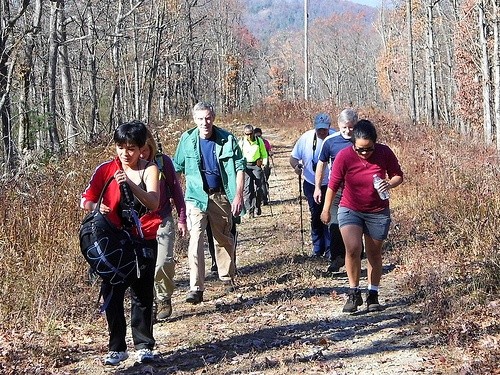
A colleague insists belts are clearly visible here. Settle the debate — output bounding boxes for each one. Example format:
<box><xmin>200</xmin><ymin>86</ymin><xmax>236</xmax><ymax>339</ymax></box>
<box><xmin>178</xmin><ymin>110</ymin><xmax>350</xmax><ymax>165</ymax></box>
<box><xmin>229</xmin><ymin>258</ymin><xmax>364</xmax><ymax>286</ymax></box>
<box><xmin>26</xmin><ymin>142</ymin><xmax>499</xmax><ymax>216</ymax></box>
<box><xmin>204</xmin><ymin>188</ymin><xmax>224</xmax><ymax>194</ymax></box>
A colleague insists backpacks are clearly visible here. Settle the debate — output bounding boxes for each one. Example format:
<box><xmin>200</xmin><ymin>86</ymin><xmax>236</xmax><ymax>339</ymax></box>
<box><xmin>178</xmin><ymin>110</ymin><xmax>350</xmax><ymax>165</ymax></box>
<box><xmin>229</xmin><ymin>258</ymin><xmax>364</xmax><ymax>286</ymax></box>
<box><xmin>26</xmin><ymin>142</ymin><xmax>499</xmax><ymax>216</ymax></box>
<box><xmin>78</xmin><ymin>173</ymin><xmax>151</xmax><ymax>298</ymax></box>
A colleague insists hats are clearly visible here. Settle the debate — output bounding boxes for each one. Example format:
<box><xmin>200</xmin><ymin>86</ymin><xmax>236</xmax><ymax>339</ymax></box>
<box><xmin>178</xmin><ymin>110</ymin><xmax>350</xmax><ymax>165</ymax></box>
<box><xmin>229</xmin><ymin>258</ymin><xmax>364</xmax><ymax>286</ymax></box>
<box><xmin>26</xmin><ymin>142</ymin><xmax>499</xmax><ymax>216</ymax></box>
<box><xmin>314</xmin><ymin>112</ymin><xmax>332</xmax><ymax>130</ymax></box>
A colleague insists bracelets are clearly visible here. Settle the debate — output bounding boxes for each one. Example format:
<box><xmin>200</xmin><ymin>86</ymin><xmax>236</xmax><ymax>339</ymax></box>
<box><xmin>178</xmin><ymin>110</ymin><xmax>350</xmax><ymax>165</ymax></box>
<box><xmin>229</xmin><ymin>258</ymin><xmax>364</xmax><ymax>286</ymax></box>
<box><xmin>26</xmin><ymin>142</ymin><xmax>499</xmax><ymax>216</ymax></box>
<box><xmin>293</xmin><ymin>163</ymin><xmax>298</xmax><ymax>168</ymax></box>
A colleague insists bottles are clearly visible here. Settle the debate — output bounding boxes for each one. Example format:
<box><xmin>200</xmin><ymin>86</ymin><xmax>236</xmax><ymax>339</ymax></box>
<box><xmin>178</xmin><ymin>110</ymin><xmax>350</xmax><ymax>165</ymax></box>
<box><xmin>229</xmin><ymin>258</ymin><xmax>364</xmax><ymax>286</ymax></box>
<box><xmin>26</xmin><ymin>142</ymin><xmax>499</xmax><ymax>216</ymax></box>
<box><xmin>119</xmin><ymin>182</ymin><xmax>134</xmax><ymax>204</ymax></box>
<box><xmin>371</xmin><ymin>173</ymin><xmax>391</xmax><ymax>200</ymax></box>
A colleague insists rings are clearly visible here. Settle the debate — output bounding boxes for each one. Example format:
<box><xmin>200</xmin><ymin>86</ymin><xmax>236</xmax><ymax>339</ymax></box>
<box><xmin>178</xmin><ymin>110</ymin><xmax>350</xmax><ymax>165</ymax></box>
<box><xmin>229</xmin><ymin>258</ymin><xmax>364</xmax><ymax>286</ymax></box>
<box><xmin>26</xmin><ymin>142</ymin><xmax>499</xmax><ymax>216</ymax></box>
<box><xmin>240</xmin><ymin>210</ymin><xmax>242</xmax><ymax>212</ymax></box>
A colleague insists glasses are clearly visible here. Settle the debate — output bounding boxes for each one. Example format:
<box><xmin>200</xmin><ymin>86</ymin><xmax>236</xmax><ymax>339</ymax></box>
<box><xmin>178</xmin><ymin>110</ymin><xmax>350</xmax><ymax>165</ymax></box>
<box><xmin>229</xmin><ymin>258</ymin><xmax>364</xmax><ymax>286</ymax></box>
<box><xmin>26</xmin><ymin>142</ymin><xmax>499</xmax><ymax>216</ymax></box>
<box><xmin>259</xmin><ymin>134</ymin><xmax>262</xmax><ymax>138</ymax></box>
<box><xmin>245</xmin><ymin>131</ymin><xmax>253</xmax><ymax>136</ymax></box>
<box><xmin>353</xmin><ymin>140</ymin><xmax>375</xmax><ymax>153</ymax></box>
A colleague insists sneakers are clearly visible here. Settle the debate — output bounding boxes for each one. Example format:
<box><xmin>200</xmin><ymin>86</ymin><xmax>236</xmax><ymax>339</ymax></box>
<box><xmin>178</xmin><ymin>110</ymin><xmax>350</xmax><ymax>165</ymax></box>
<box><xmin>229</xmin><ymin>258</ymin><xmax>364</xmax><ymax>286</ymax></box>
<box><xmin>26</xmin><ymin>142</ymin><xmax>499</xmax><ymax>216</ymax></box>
<box><xmin>342</xmin><ymin>289</ymin><xmax>363</xmax><ymax>313</ymax></box>
<box><xmin>155</xmin><ymin>300</ymin><xmax>172</xmax><ymax>320</ymax></box>
<box><xmin>105</xmin><ymin>349</ymin><xmax>129</xmax><ymax>366</ymax></box>
<box><xmin>152</xmin><ymin>307</ymin><xmax>158</xmax><ymax>325</ymax></box>
<box><xmin>365</xmin><ymin>289</ymin><xmax>381</xmax><ymax>312</ymax></box>
<box><xmin>327</xmin><ymin>256</ymin><xmax>345</xmax><ymax>272</ymax></box>
<box><xmin>136</xmin><ymin>348</ymin><xmax>154</xmax><ymax>362</ymax></box>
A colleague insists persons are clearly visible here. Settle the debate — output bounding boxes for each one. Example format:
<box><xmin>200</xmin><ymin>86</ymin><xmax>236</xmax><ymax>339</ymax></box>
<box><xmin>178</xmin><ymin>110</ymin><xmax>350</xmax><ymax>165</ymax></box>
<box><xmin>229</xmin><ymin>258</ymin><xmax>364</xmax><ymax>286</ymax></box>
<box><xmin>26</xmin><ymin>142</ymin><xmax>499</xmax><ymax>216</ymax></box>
<box><xmin>78</xmin><ymin>119</ymin><xmax>160</xmax><ymax>366</ymax></box>
<box><xmin>287</xmin><ymin>114</ymin><xmax>340</xmax><ymax>260</ymax></box>
<box><xmin>138</xmin><ymin>129</ymin><xmax>188</xmax><ymax>324</ymax></box>
<box><xmin>237</xmin><ymin>121</ymin><xmax>273</xmax><ymax>220</ymax></box>
<box><xmin>169</xmin><ymin>101</ymin><xmax>247</xmax><ymax>302</ymax></box>
<box><xmin>319</xmin><ymin>119</ymin><xmax>405</xmax><ymax>312</ymax></box>
<box><xmin>313</xmin><ymin>108</ymin><xmax>359</xmax><ymax>272</ymax></box>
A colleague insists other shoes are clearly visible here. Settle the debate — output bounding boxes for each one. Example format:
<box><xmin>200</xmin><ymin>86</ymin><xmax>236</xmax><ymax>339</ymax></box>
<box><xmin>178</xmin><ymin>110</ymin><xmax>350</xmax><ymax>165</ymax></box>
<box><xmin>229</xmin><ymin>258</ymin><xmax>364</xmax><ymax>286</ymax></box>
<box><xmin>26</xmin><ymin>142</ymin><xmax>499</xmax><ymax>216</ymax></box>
<box><xmin>261</xmin><ymin>200</ymin><xmax>269</xmax><ymax>206</ymax></box>
<box><xmin>256</xmin><ymin>207</ymin><xmax>261</xmax><ymax>215</ymax></box>
<box><xmin>223</xmin><ymin>278</ymin><xmax>236</xmax><ymax>296</ymax></box>
<box><xmin>310</xmin><ymin>252</ymin><xmax>323</xmax><ymax>260</ymax></box>
<box><xmin>185</xmin><ymin>290</ymin><xmax>204</xmax><ymax>305</ymax></box>
<box><xmin>249</xmin><ymin>212</ymin><xmax>255</xmax><ymax>219</ymax></box>
